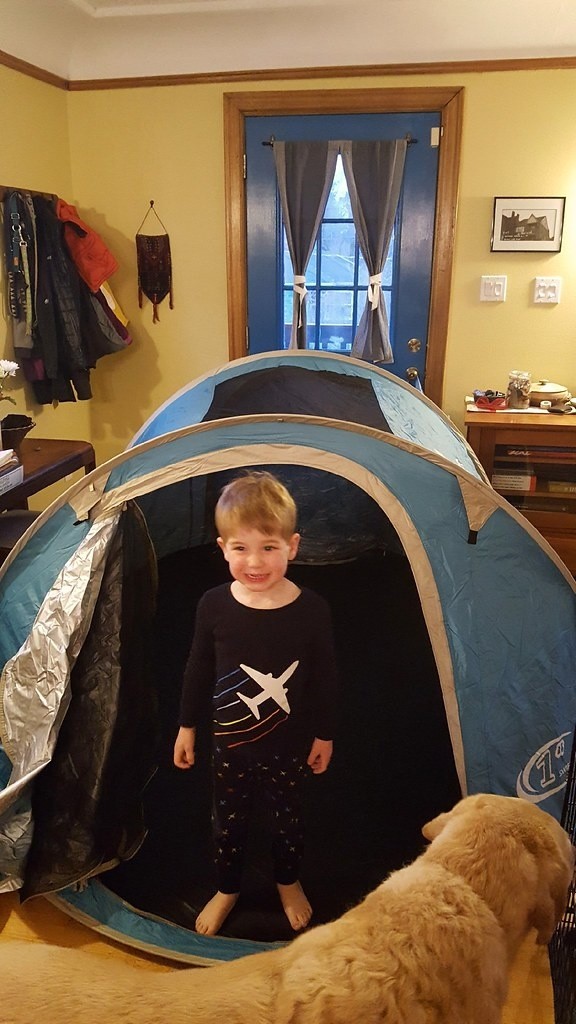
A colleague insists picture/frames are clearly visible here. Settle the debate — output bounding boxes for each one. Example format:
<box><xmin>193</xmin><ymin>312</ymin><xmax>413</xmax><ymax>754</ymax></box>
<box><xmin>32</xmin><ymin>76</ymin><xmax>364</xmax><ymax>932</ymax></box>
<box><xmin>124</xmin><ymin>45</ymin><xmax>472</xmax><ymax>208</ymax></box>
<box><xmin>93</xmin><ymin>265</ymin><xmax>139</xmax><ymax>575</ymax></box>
<box><xmin>489</xmin><ymin>197</ymin><xmax>566</xmax><ymax>252</ymax></box>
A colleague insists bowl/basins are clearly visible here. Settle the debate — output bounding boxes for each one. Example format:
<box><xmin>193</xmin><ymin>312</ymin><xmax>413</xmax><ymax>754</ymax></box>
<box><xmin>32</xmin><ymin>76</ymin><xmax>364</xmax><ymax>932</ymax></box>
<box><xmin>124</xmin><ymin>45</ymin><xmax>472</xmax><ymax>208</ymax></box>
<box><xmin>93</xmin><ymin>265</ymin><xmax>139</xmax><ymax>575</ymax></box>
<box><xmin>473</xmin><ymin>391</ymin><xmax>509</xmax><ymax>409</ymax></box>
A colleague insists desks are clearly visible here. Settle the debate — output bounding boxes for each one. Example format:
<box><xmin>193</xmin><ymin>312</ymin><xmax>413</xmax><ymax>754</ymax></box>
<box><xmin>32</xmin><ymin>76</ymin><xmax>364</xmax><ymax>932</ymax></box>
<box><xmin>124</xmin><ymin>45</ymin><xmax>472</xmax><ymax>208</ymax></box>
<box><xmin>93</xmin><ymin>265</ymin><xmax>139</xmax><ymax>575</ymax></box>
<box><xmin>0</xmin><ymin>438</ymin><xmax>97</xmax><ymax>515</ymax></box>
<box><xmin>463</xmin><ymin>401</ymin><xmax>576</xmax><ymax>580</ymax></box>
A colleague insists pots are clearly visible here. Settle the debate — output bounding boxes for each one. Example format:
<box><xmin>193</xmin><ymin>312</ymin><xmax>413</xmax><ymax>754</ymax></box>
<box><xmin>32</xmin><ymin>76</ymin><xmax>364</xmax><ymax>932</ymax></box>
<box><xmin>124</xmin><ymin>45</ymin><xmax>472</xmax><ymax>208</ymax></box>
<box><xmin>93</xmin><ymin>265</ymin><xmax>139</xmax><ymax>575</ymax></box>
<box><xmin>529</xmin><ymin>380</ymin><xmax>568</xmax><ymax>407</ymax></box>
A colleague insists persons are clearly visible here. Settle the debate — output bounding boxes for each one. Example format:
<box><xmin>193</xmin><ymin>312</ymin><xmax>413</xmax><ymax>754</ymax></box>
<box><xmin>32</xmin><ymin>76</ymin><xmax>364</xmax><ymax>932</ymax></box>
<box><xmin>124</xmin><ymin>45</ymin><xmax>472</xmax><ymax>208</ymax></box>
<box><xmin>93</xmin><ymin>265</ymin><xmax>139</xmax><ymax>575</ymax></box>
<box><xmin>173</xmin><ymin>472</ymin><xmax>341</xmax><ymax>935</ymax></box>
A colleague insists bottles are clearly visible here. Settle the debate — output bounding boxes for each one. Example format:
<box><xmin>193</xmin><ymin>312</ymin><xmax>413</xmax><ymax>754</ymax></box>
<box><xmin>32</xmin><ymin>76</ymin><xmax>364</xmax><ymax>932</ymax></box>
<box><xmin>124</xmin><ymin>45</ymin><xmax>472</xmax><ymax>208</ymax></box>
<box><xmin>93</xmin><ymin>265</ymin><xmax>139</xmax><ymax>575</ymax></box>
<box><xmin>508</xmin><ymin>371</ymin><xmax>531</xmax><ymax>408</ymax></box>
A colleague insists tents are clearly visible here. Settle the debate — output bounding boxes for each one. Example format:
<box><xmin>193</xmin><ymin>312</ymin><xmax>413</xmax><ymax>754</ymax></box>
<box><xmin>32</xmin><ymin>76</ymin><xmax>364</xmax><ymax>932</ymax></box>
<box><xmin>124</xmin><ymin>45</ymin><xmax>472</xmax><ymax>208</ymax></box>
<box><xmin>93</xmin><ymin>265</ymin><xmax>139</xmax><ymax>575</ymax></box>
<box><xmin>0</xmin><ymin>348</ymin><xmax>576</xmax><ymax>968</ymax></box>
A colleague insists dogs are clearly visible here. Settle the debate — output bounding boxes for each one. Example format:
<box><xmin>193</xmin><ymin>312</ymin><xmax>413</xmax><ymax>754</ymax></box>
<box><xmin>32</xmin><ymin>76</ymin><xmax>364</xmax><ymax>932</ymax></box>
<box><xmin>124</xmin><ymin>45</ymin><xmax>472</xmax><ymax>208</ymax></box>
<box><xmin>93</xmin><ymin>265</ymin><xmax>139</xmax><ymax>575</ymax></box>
<box><xmin>0</xmin><ymin>794</ymin><xmax>576</xmax><ymax>1022</ymax></box>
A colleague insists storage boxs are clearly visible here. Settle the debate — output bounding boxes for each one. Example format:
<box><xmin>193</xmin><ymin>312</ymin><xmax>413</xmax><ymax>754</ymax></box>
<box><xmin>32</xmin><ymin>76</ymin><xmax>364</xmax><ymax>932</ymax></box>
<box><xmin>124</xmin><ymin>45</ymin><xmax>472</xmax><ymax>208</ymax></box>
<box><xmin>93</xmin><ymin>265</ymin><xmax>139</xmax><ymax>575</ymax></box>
<box><xmin>0</xmin><ymin>464</ymin><xmax>23</xmax><ymax>497</ymax></box>
